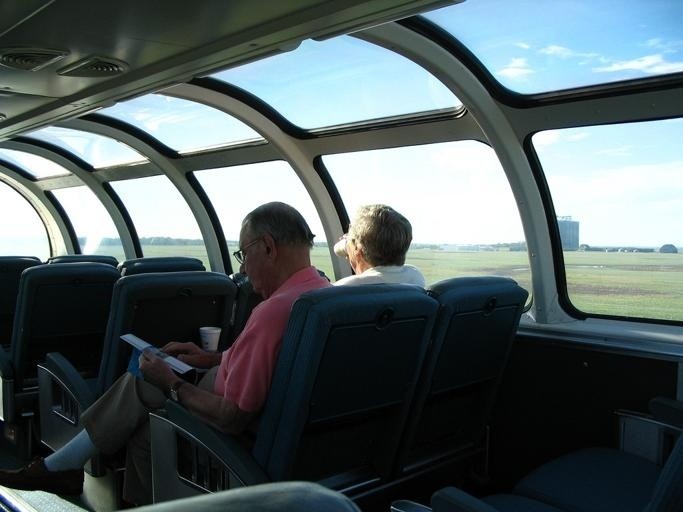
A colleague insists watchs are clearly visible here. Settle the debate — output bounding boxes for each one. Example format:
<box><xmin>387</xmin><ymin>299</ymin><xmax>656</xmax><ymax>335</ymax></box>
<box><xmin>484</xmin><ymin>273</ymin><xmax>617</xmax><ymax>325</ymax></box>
<box><xmin>170</xmin><ymin>379</ymin><xmax>188</xmax><ymax>407</ymax></box>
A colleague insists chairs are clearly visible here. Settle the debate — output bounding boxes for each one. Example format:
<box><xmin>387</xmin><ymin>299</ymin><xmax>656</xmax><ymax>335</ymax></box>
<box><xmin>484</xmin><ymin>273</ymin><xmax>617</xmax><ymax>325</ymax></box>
<box><xmin>146</xmin><ymin>276</ymin><xmax>530</xmax><ymax>510</ymax></box>
<box><xmin>35</xmin><ymin>270</ymin><xmax>328</xmax><ymax>510</ymax></box>
<box><xmin>431</xmin><ymin>396</ymin><xmax>680</xmax><ymax>511</ymax></box>
<box><xmin>1</xmin><ymin>258</ymin><xmax>208</xmax><ymax>462</ymax></box>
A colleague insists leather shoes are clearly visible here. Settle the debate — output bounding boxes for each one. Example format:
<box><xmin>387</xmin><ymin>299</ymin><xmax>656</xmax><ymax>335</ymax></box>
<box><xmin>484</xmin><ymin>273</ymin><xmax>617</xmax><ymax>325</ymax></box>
<box><xmin>0</xmin><ymin>456</ymin><xmax>84</xmax><ymax>496</ymax></box>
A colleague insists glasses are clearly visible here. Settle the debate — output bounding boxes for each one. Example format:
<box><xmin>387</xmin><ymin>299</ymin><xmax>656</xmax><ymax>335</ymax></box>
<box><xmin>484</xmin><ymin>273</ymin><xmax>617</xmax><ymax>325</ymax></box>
<box><xmin>233</xmin><ymin>237</ymin><xmax>259</xmax><ymax>265</ymax></box>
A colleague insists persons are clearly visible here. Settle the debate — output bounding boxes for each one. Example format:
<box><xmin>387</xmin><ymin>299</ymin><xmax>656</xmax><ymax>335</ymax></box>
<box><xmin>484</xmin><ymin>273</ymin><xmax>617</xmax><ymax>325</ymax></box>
<box><xmin>332</xmin><ymin>203</ymin><xmax>426</xmax><ymax>289</ymax></box>
<box><xmin>0</xmin><ymin>202</ymin><xmax>333</xmax><ymax>509</ymax></box>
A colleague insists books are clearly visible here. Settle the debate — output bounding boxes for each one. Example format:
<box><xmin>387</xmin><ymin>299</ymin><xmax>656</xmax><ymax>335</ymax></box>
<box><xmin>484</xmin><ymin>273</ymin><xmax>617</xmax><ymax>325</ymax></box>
<box><xmin>120</xmin><ymin>333</ymin><xmax>208</xmax><ymax>386</ymax></box>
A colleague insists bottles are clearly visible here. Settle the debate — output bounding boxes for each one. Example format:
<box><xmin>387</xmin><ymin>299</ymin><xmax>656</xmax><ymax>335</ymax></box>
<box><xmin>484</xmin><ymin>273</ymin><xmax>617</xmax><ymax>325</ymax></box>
<box><xmin>229</xmin><ymin>271</ymin><xmax>248</xmax><ymax>288</ymax></box>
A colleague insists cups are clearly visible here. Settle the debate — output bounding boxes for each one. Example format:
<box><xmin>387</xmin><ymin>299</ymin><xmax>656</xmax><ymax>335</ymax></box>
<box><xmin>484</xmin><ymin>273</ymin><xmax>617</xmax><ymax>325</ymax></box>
<box><xmin>333</xmin><ymin>238</ymin><xmax>349</xmax><ymax>258</ymax></box>
<box><xmin>200</xmin><ymin>325</ymin><xmax>221</xmax><ymax>351</ymax></box>
